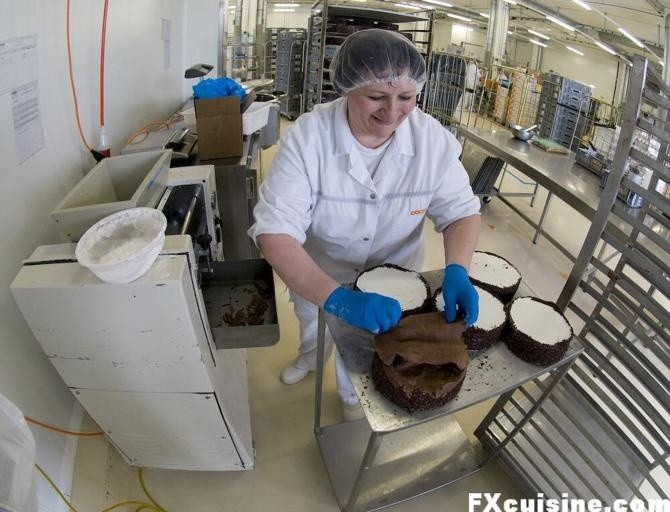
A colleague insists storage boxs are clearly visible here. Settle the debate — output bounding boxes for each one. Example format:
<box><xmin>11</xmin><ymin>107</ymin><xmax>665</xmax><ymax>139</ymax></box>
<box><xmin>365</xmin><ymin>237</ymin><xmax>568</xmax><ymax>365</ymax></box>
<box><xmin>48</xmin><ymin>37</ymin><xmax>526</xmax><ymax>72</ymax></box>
<box><xmin>193</xmin><ymin>88</ymin><xmax>256</xmax><ymax>161</ymax></box>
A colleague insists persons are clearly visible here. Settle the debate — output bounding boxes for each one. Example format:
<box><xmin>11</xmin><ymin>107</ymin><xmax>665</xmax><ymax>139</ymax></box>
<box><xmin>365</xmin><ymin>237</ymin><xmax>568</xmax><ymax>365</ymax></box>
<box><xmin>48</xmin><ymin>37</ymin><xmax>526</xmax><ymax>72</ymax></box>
<box><xmin>247</xmin><ymin>29</ymin><xmax>483</xmax><ymax>422</ymax></box>
<box><xmin>464</xmin><ymin>58</ymin><xmax>481</xmax><ymax>111</ymax></box>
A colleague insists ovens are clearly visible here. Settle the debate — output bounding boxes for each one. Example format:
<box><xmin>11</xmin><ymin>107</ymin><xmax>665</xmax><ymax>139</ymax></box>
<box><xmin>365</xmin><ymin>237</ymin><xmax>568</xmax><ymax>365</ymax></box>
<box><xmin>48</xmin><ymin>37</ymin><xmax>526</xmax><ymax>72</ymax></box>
<box><xmin>22</xmin><ymin>164</ymin><xmax>225</xmax><ymax>393</ymax></box>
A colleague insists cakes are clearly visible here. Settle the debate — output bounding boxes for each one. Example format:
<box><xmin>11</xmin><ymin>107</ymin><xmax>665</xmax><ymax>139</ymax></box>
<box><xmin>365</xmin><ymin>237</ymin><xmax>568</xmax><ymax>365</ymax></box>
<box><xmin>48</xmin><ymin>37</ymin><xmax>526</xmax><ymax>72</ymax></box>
<box><xmin>353</xmin><ymin>250</ymin><xmax>575</xmax><ymax>412</ymax></box>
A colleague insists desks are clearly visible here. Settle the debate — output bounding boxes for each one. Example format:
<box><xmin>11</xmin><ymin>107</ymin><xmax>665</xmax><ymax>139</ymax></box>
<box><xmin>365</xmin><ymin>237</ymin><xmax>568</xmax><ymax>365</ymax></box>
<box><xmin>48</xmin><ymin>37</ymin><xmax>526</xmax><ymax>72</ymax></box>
<box><xmin>460</xmin><ymin>128</ymin><xmax>669</xmax><ymax>293</ymax></box>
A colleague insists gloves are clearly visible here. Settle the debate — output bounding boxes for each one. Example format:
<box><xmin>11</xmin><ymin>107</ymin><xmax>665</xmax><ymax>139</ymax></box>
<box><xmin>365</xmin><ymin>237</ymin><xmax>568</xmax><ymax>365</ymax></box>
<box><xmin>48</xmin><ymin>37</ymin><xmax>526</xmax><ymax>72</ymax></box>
<box><xmin>443</xmin><ymin>263</ymin><xmax>478</xmax><ymax>325</ymax></box>
<box><xmin>324</xmin><ymin>287</ymin><xmax>401</xmax><ymax>334</ymax></box>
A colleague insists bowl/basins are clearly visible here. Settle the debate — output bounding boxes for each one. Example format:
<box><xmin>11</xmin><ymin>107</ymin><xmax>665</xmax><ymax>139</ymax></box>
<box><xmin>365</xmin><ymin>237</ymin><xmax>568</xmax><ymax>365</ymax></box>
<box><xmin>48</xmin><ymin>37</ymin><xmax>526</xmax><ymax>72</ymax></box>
<box><xmin>75</xmin><ymin>206</ymin><xmax>168</xmax><ymax>284</ymax></box>
<box><xmin>510</xmin><ymin>123</ymin><xmax>536</xmax><ymax>140</ymax></box>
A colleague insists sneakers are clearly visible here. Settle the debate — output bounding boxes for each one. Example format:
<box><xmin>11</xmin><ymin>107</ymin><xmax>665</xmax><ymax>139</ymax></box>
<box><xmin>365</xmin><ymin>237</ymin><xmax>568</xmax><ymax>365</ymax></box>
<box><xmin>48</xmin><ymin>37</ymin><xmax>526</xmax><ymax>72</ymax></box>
<box><xmin>338</xmin><ymin>391</ymin><xmax>359</xmax><ymax>406</ymax></box>
<box><xmin>281</xmin><ymin>365</ymin><xmax>308</xmax><ymax>385</ymax></box>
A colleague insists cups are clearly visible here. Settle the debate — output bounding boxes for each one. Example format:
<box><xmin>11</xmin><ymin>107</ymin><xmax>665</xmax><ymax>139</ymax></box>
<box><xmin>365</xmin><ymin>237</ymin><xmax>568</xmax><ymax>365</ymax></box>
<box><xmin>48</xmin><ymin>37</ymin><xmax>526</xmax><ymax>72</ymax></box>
<box><xmin>627</xmin><ymin>171</ymin><xmax>642</xmax><ymax>185</ymax></box>
